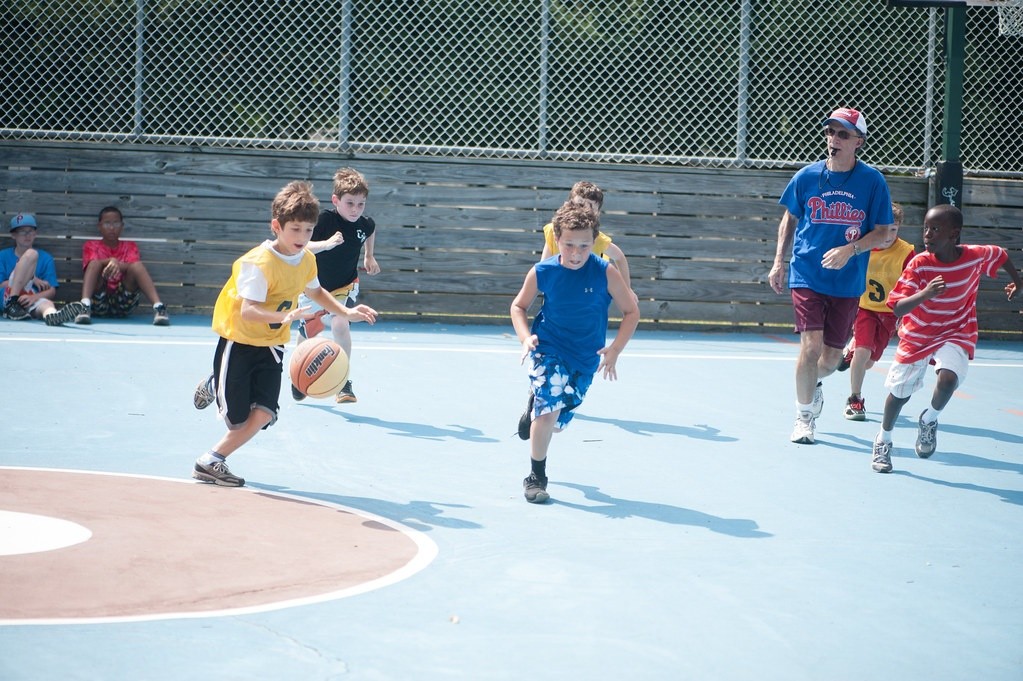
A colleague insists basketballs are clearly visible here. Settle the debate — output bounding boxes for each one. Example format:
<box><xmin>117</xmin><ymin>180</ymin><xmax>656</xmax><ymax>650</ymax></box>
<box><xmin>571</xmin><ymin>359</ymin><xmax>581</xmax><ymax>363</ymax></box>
<box><xmin>289</xmin><ymin>338</ymin><xmax>349</xmax><ymax>399</ymax></box>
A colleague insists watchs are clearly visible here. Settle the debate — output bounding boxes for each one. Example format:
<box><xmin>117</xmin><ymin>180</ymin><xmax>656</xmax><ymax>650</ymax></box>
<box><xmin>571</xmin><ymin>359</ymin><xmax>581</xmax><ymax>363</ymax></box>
<box><xmin>851</xmin><ymin>241</ymin><xmax>861</xmax><ymax>256</ymax></box>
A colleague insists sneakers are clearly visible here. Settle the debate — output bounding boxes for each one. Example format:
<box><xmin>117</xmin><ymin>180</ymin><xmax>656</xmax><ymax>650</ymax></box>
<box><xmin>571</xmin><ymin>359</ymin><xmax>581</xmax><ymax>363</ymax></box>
<box><xmin>836</xmin><ymin>336</ymin><xmax>854</xmax><ymax>372</ymax></box>
<box><xmin>513</xmin><ymin>393</ymin><xmax>534</xmax><ymax>441</ymax></box>
<box><xmin>790</xmin><ymin>410</ymin><xmax>816</xmax><ymax>444</ymax></box>
<box><xmin>192</xmin><ymin>458</ymin><xmax>245</xmax><ymax>487</ymax></box>
<box><xmin>152</xmin><ymin>302</ymin><xmax>169</xmax><ymax>325</ymax></box>
<box><xmin>335</xmin><ymin>379</ymin><xmax>357</xmax><ymax>403</ymax></box>
<box><xmin>844</xmin><ymin>394</ymin><xmax>866</xmax><ymax>420</ymax></box>
<box><xmin>871</xmin><ymin>432</ymin><xmax>893</xmax><ymax>473</ymax></box>
<box><xmin>522</xmin><ymin>471</ymin><xmax>550</xmax><ymax>503</ymax></box>
<box><xmin>74</xmin><ymin>302</ymin><xmax>93</xmax><ymax>324</ymax></box>
<box><xmin>193</xmin><ymin>369</ymin><xmax>216</xmax><ymax>410</ymax></box>
<box><xmin>45</xmin><ymin>302</ymin><xmax>84</xmax><ymax>326</ymax></box>
<box><xmin>3</xmin><ymin>301</ymin><xmax>32</xmax><ymax>321</ymax></box>
<box><xmin>914</xmin><ymin>408</ymin><xmax>938</xmax><ymax>459</ymax></box>
<box><xmin>810</xmin><ymin>381</ymin><xmax>824</xmax><ymax>418</ymax></box>
<box><xmin>292</xmin><ymin>383</ymin><xmax>307</xmax><ymax>401</ymax></box>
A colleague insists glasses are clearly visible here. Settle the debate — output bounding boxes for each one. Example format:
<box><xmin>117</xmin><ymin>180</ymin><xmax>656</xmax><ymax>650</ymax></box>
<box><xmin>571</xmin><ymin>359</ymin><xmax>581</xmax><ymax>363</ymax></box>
<box><xmin>98</xmin><ymin>220</ymin><xmax>122</xmax><ymax>229</ymax></box>
<box><xmin>14</xmin><ymin>229</ymin><xmax>36</xmax><ymax>236</ymax></box>
<box><xmin>823</xmin><ymin>128</ymin><xmax>859</xmax><ymax>139</ymax></box>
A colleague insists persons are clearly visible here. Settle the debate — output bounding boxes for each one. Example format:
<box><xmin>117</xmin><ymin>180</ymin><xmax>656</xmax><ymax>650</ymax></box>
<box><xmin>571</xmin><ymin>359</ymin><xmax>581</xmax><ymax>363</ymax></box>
<box><xmin>0</xmin><ymin>213</ymin><xmax>83</xmax><ymax>325</ymax></box>
<box><xmin>835</xmin><ymin>203</ymin><xmax>917</xmax><ymax>421</ymax></box>
<box><xmin>767</xmin><ymin>107</ymin><xmax>895</xmax><ymax>444</ymax></box>
<box><xmin>74</xmin><ymin>206</ymin><xmax>170</xmax><ymax>325</ymax></box>
<box><xmin>872</xmin><ymin>204</ymin><xmax>1023</xmax><ymax>475</ymax></box>
<box><xmin>509</xmin><ymin>198</ymin><xmax>642</xmax><ymax>503</ymax></box>
<box><xmin>540</xmin><ymin>182</ymin><xmax>639</xmax><ymax>306</ymax></box>
<box><xmin>192</xmin><ymin>179</ymin><xmax>379</xmax><ymax>488</ymax></box>
<box><xmin>292</xmin><ymin>166</ymin><xmax>380</xmax><ymax>404</ymax></box>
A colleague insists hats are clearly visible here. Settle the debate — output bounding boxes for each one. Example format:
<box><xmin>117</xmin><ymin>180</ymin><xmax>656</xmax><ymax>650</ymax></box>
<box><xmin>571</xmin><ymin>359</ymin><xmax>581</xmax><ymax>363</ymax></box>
<box><xmin>9</xmin><ymin>214</ymin><xmax>41</xmax><ymax>232</ymax></box>
<box><xmin>822</xmin><ymin>107</ymin><xmax>867</xmax><ymax>134</ymax></box>
